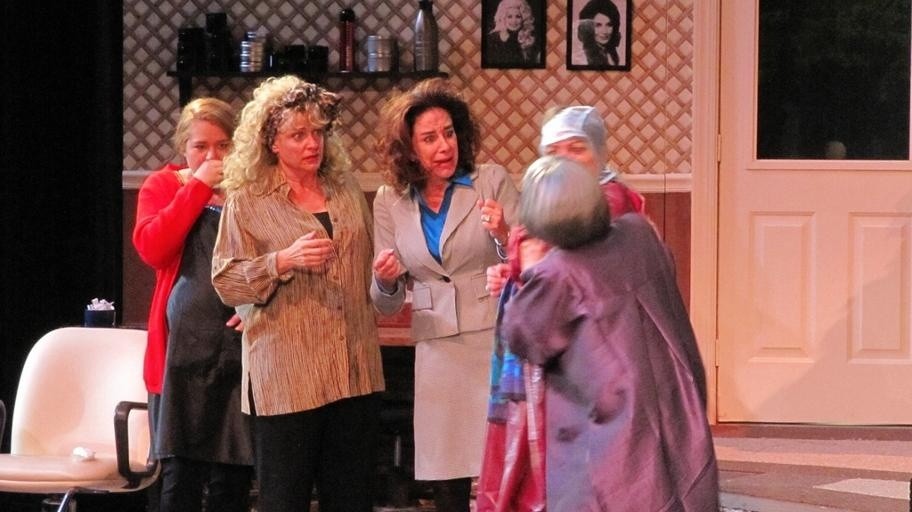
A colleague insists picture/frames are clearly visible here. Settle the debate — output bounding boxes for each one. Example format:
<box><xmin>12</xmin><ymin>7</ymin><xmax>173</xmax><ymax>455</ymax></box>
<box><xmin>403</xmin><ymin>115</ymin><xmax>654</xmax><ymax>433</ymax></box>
<box><xmin>564</xmin><ymin>0</ymin><xmax>633</xmax><ymax>74</ymax></box>
<box><xmin>479</xmin><ymin>0</ymin><xmax>548</xmax><ymax>72</ymax></box>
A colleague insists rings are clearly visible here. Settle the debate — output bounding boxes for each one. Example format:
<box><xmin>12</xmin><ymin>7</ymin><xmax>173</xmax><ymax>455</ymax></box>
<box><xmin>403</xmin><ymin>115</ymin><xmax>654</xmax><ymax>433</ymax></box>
<box><xmin>486</xmin><ymin>214</ymin><xmax>493</xmax><ymax>223</ymax></box>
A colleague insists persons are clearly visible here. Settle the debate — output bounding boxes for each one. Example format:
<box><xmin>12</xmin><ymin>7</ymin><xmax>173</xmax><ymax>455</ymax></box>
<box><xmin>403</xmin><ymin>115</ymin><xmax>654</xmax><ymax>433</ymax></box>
<box><xmin>500</xmin><ymin>155</ymin><xmax>718</xmax><ymax>511</ymax></box>
<box><xmin>210</xmin><ymin>74</ymin><xmax>388</xmax><ymax>512</ymax></box>
<box><xmin>484</xmin><ymin>1</ymin><xmax>541</xmax><ymax>64</ymax></box>
<box><xmin>574</xmin><ymin>0</ymin><xmax>622</xmax><ymax>66</ymax></box>
<box><xmin>368</xmin><ymin>81</ymin><xmax>524</xmax><ymax>512</ymax></box>
<box><xmin>486</xmin><ymin>105</ymin><xmax>645</xmax><ymax>510</ymax></box>
<box><xmin>132</xmin><ymin>98</ymin><xmax>263</xmax><ymax>510</ymax></box>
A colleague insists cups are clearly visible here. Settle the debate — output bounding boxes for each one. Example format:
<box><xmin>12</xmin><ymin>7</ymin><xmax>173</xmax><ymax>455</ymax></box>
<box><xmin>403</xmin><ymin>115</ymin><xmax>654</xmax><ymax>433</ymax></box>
<box><xmin>366</xmin><ymin>34</ymin><xmax>398</xmax><ymax>72</ymax></box>
<box><xmin>235</xmin><ymin>32</ymin><xmax>269</xmax><ymax>70</ymax></box>
<box><xmin>282</xmin><ymin>44</ymin><xmax>327</xmax><ymax>67</ymax></box>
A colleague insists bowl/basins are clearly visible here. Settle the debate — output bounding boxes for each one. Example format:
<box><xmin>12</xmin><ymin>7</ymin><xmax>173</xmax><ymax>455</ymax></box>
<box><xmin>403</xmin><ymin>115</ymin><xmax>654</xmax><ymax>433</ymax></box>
<box><xmin>83</xmin><ymin>310</ymin><xmax>117</xmax><ymax>327</ymax></box>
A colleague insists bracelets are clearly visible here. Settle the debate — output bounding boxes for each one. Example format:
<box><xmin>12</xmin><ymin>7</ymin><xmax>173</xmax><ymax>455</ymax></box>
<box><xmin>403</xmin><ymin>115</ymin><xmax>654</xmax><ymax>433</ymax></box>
<box><xmin>493</xmin><ymin>232</ymin><xmax>510</xmax><ymax>247</ymax></box>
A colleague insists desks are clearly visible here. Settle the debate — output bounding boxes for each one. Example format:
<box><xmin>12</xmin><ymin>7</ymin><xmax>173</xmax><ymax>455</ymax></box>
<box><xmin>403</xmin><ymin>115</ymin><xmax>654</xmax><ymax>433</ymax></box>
<box><xmin>377</xmin><ymin>324</ymin><xmax>416</xmax><ymax>500</ymax></box>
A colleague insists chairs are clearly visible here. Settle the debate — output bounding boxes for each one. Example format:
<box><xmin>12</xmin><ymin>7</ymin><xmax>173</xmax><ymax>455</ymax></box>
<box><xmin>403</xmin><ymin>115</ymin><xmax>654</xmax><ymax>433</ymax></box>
<box><xmin>1</xmin><ymin>323</ymin><xmax>163</xmax><ymax>512</ymax></box>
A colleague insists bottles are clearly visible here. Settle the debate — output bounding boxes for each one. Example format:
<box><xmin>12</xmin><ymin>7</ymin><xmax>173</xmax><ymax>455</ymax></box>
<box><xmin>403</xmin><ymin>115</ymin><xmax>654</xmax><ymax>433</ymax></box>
<box><xmin>412</xmin><ymin>1</ymin><xmax>441</xmax><ymax>73</ymax></box>
<box><xmin>177</xmin><ymin>10</ymin><xmax>232</xmax><ymax>74</ymax></box>
<box><xmin>338</xmin><ymin>7</ymin><xmax>357</xmax><ymax>74</ymax></box>
